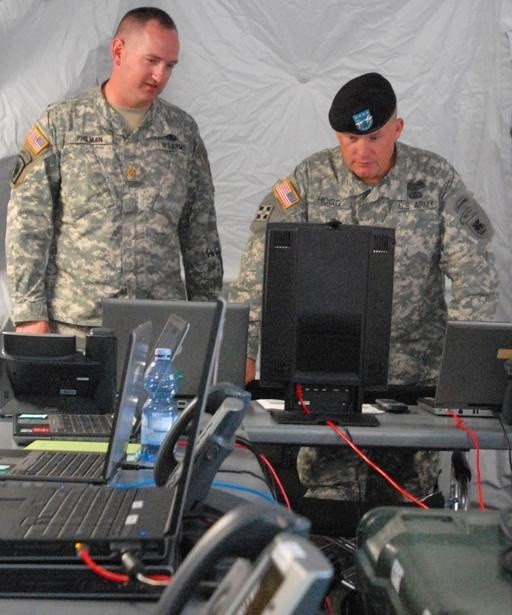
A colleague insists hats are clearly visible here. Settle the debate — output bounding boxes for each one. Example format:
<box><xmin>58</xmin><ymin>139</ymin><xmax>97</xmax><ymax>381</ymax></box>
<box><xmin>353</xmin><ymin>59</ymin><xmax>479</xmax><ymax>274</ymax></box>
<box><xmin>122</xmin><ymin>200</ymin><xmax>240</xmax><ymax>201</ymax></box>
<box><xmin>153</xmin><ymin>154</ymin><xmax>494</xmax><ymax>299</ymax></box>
<box><xmin>327</xmin><ymin>71</ymin><xmax>399</xmax><ymax>136</ymax></box>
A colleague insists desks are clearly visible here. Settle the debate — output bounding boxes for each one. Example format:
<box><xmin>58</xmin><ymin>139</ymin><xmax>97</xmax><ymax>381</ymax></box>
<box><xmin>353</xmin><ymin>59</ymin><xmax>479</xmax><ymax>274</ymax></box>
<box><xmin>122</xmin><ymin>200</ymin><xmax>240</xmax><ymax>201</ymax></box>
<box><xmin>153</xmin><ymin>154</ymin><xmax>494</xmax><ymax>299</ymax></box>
<box><xmin>0</xmin><ymin>397</ymin><xmax>277</xmax><ymax>615</ymax></box>
<box><xmin>243</xmin><ymin>396</ymin><xmax>512</xmax><ymax>451</ymax></box>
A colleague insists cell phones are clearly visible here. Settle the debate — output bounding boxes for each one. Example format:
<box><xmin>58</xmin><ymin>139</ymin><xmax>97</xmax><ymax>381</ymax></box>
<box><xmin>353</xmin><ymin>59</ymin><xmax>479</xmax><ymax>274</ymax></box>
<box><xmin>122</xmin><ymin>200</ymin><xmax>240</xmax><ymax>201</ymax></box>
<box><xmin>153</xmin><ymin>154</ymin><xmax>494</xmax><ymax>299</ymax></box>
<box><xmin>375</xmin><ymin>398</ymin><xmax>408</xmax><ymax>413</ymax></box>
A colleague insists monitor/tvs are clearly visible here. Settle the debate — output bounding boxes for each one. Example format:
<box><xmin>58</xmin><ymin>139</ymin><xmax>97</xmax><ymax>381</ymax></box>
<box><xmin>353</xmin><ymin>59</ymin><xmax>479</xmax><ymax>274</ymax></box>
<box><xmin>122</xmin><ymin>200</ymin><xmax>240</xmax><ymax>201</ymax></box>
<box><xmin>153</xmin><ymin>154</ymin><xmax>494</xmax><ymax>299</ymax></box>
<box><xmin>259</xmin><ymin>223</ymin><xmax>394</xmax><ymax>426</ymax></box>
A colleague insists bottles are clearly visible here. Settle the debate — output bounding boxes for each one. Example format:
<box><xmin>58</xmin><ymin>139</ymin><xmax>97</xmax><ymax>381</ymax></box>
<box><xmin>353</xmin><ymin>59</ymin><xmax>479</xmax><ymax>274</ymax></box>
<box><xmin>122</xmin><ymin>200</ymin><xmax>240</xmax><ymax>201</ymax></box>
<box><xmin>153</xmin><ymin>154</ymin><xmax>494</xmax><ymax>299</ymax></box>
<box><xmin>136</xmin><ymin>347</ymin><xmax>188</xmax><ymax>470</ymax></box>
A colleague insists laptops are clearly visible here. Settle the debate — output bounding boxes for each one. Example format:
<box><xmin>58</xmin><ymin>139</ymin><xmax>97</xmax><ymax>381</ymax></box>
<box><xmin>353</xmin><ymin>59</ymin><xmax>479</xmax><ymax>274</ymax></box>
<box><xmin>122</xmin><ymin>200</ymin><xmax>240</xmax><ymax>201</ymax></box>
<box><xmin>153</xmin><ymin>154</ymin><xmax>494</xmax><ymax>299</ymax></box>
<box><xmin>417</xmin><ymin>321</ymin><xmax>512</xmax><ymax>419</ymax></box>
<box><xmin>1</xmin><ymin>300</ymin><xmax>226</xmax><ymax>562</ymax></box>
<box><xmin>12</xmin><ymin>310</ymin><xmax>189</xmax><ymax>444</ymax></box>
<box><xmin>102</xmin><ymin>299</ymin><xmax>249</xmax><ymax>409</ymax></box>
<box><xmin>0</xmin><ymin>318</ymin><xmax>152</xmax><ymax>486</ymax></box>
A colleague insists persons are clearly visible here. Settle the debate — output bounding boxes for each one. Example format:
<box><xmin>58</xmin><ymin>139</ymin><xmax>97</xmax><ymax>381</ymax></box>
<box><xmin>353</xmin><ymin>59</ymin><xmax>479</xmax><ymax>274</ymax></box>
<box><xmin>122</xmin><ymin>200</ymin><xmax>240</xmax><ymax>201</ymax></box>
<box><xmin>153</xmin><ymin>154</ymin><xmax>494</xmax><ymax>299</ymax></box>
<box><xmin>225</xmin><ymin>70</ymin><xmax>502</xmax><ymax>511</ymax></box>
<box><xmin>5</xmin><ymin>3</ymin><xmax>224</xmax><ymax>357</ymax></box>
